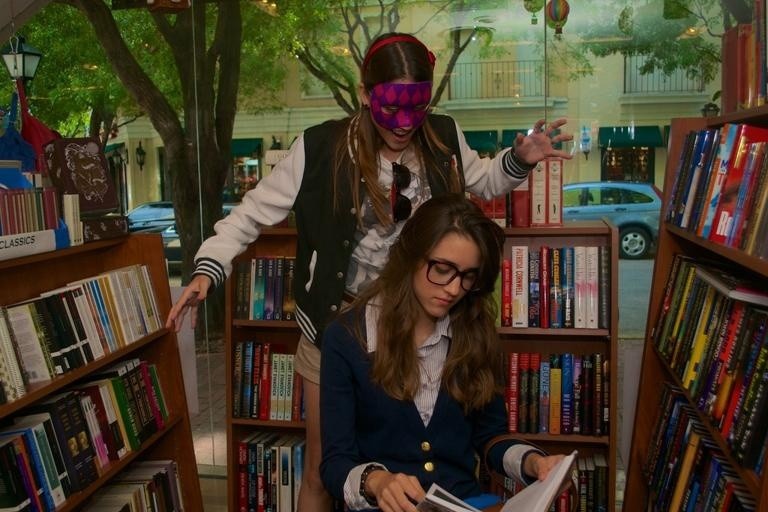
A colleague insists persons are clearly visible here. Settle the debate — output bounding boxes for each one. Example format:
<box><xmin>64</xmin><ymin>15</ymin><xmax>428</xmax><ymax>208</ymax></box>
<box><xmin>320</xmin><ymin>191</ymin><xmax>574</xmax><ymax>512</ymax></box>
<box><xmin>166</xmin><ymin>33</ymin><xmax>573</xmax><ymax>511</ymax></box>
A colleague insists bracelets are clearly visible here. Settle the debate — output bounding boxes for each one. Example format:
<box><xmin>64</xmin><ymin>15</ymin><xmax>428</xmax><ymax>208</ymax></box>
<box><xmin>360</xmin><ymin>460</ymin><xmax>378</xmax><ymax>508</ymax></box>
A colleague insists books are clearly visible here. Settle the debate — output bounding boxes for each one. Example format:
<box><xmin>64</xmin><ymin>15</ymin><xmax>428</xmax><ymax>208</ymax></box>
<box><xmin>465</xmin><ymin>151</ymin><xmax>563</xmax><ymax>229</ymax></box>
<box><xmin>718</xmin><ymin>1</ymin><xmax>767</xmax><ymax>115</ymax></box>
<box><xmin>231</xmin><ymin>257</ymin><xmax>311</xmax><ymax>511</ymax></box>
<box><xmin>484</xmin><ymin>243</ymin><xmax>609</xmax><ymax>512</ymax></box>
<box><xmin>409</xmin><ymin>445</ymin><xmax>579</xmax><ymax>512</ymax></box>
<box><xmin>0</xmin><ymin>185</ymin><xmax>85</xmax><ymax>257</ymax></box>
<box><xmin>45</xmin><ymin>135</ymin><xmax>121</xmax><ymax>215</ymax></box>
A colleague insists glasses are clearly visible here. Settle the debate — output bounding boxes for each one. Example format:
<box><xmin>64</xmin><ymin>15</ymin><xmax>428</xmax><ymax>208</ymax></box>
<box><xmin>425</xmin><ymin>257</ymin><xmax>484</xmax><ymax>292</ymax></box>
<box><xmin>392</xmin><ymin>161</ymin><xmax>412</xmax><ymax>222</ymax></box>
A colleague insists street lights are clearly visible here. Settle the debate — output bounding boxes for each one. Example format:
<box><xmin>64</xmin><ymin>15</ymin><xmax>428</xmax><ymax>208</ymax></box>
<box><xmin>135</xmin><ymin>140</ymin><xmax>145</xmax><ymax>204</ymax></box>
<box><xmin>1</xmin><ymin>34</ymin><xmax>41</xmax><ymax>101</ymax></box>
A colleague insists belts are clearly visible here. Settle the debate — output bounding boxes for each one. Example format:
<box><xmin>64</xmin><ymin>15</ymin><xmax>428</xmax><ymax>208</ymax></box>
<box><xmin>342</xmin><ymin>292</ymin><xmax>354</xmax><ymax>303</ymax></box>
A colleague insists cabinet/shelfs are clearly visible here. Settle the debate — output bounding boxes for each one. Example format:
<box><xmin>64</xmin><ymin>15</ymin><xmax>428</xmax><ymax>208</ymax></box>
<box><xmin>1</xmin><ymin>233</ymin><xmax>205</xmax><ymax>512</ymax></box>
<box><xmin>621</xmin><ymin>104</ymin><xmax>768</xmax><ymax>512</ymax></box>
<box><xmin>225</xmin><ymin>218</ymin><xmax>619</xmax><ymax>512</ymax></box>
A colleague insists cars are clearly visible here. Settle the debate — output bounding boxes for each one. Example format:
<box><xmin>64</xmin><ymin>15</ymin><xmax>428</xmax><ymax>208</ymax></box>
<box><xmin>563</xmin><ymin>180</ymin><xmax>662</xmax><ymax>258</ymax></box>
<box><xmin>126</xmin><ymin>200</ymin><xmax>237</xmax><ymax>271</ymax></box>
